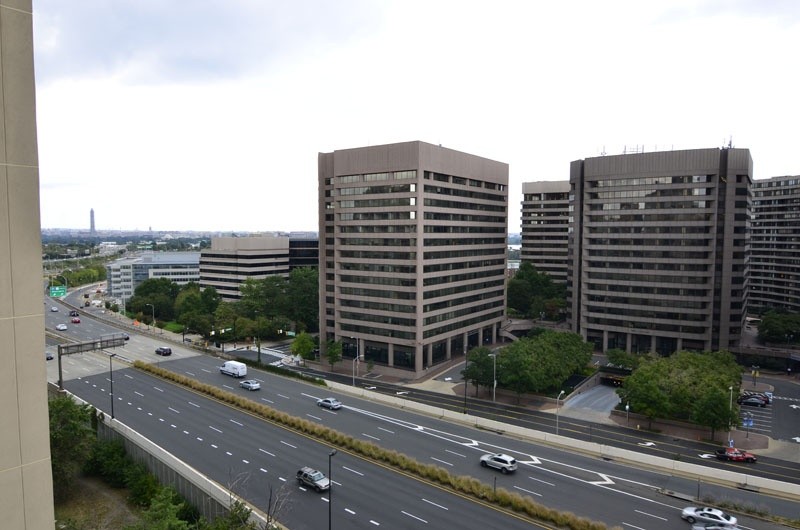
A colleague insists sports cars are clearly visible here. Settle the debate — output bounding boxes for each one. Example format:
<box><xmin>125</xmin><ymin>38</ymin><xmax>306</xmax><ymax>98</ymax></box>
<box><xmin>715</xmin><ymin>446</ymin><xmax>757</xmax><ymax>463</ymax></box>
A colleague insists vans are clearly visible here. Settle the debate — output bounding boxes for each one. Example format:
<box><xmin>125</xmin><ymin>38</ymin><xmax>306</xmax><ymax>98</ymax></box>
<box><xmin>220</xmin><ymin>359</ymin><xmax>247</xmax><ymax>378</ymax></box>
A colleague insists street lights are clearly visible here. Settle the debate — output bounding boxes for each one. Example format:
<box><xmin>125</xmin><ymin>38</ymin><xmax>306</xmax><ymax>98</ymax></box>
<box><xmin>109</xmin><ymin>352</ymin><xmax>116</xmax><ymax>419</ymax></box>
<box><xmin>555</xmin><ymin>389</ymin><xmax>566</xmax><ymax>436</ymax></box>
<box><xmin>352</xmin><ymin>354</ymin><xmax>364</xmax><ymax>387</ymax></box>
<box><xmin>146</xmin><ymin>303</ymin><xmax>155</xmax><ymax>336</ymax></box>
<box><xmin>728</xmin><ymin>385</ymin><xmax>733</xmax><ymax>444</ymax></box>
<box><xmin>351</xmin><ymin>335</ymin><xmax>359</xmax><ymax>378</ymax></box>
<box><xmin>327</xmin><ymin>448</ymin><xmax>338</xmax><ymax>530</ymax></box>
<box><xmin>488</xmin><ymin>353</ymin><xmax>497</xmax><ymax>402</ymax></box>
<box><xmin>625</xmin><ymin>400</ymin><xmax>630</xmax><ymax>427</ymax></box>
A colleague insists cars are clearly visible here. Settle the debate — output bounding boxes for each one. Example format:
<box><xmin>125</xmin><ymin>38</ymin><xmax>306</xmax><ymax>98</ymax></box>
<box><xmin>45</xmin><ymin>351</ymin><xmax>53</xmax><ymax>360</ymax></box>
<box><xmin>746</xmin><ymin>393</ymin><xmax>769</xmax><ymax>403</ymax></box>
<box><xmin>740</xmin><ymin>397</ymin><xmax>765</xmax><ymax>408</ymax></box>
<box><xmin>239</xmin><ymin>379</ymin><xmax>260</xmax><ymax>391</ymax></box>
<box><xmin>680</xmin><ymin>506</ymin><xmax>738</xmax><ymax>526</ymax></box>
<box><xmin>316</xmin><ymin>397</ymin><xmax>342</xmax><ymax>411</ymax></box>
<box><xmin>48</xmin><ymin>287</ymin><xmax>103</xmax><ymax>332</ymax></box>
<box><xmin>737</xmin><ymin>394</ymin><xmax>765</xmax><ymax>405</ymax></box>
<box><xmin>692</xmin><ymin>521</ymin><xmax>756</xmax><ymax>530</ymax></box>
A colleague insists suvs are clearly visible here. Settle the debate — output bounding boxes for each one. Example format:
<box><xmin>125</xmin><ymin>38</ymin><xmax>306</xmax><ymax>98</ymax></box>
<box><xmin>479</xmin><ymin>451</ymin><xmax>518</xmax><ymax>474</ymax></box>
<box><xmin>296</xmin><ymin>465</ymin><xmax>331</xmax><ymax>492</ymax></box>
<box><xmin>155</xmin><ymin>347</ymin><xmax>172</xmax><ymax>356</ymax></box>
